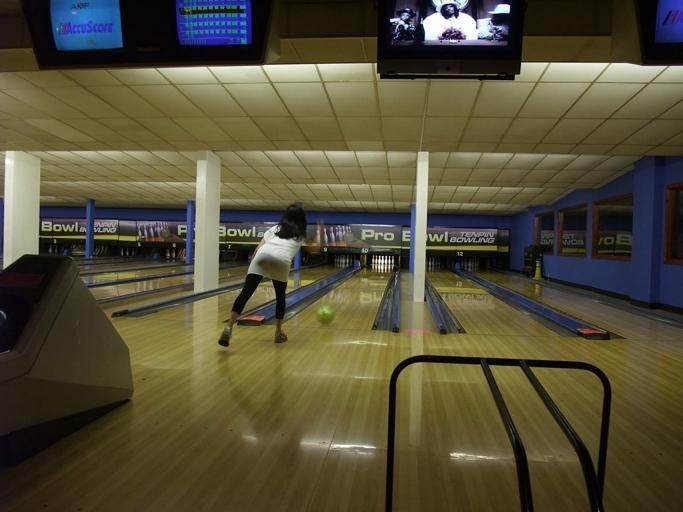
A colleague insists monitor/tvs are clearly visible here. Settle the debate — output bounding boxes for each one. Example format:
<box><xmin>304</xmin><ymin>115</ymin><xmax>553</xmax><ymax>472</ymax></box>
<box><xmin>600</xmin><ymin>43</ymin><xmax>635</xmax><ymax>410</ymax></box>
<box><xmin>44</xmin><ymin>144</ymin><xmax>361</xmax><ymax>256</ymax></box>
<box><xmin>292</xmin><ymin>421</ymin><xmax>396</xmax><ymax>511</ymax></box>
<box><xmin>637</xmin><ymin>0</ymin><xmax>683</xmax><ymax>67</ymax></box>
<box><xmin>374</xmin><ymin>0</ymin><xmax>521</xmax><ymax>83</ymax></box>
<box><xmin>25</xmin><ymin>0</ymin><xmax>132</xmax><ymax>70</ymax></box>
<box><xmin>160</xmin><ymin>0</ymin><xmax>265</xmax><ymax>65</ymax></box>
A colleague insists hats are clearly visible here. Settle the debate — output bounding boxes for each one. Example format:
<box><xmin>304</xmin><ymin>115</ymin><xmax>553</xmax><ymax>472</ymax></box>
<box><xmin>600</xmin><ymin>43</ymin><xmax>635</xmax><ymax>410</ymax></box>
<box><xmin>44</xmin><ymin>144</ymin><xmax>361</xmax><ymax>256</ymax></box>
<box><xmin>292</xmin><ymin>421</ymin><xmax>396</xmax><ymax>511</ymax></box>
<box><xmin>396</xmin><ymin>7</ymin><xmax>416</xmax><ymax>17</ymax></box>
<box><xmin>435</xmin><ymin>0</ymin><xmax>459</xmax><ymax>12</ymax></box>
<box><xmin>488</xmin><ymin>3</ymin><xmax>511</xmax><ymax>14</ymax></box>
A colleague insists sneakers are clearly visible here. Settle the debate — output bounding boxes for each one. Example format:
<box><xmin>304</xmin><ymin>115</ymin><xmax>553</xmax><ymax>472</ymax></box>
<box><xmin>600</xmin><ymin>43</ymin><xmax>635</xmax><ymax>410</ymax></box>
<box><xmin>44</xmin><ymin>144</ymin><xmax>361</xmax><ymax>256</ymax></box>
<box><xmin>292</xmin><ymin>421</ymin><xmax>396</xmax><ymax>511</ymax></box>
<box><xmin>218</xmin><ymin>326</ymin><xmax>232</xmax><ymax>347</ymax></box>
<box><xmin>275</xmin><ymin>332</ymin><xmax>288</xmax><ymax>343</ymax></box>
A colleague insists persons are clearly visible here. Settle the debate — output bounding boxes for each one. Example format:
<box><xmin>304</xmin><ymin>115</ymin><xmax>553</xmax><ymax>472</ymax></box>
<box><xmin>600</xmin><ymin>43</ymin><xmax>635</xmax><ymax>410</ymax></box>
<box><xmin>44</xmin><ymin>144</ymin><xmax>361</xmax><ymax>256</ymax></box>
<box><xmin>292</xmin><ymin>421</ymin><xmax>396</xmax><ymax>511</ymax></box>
<box><xmin>387</xmin><ymin>8</ymin><xmax>416</xmax><ymax>42</ymax></box>
<box><xmin>217</xmin><ymin>204</ymin><xmax>321</xmax><ymax>347</ymax></box>
<box><xmin>475</xmin><ymin>3</ymin><xmax>511</xmax><ymax>41</ymax></box>
<box><xmin>418</xmin><ymin>1</ymin><xmax>477</xmax><ymax>42</ymax></box>
